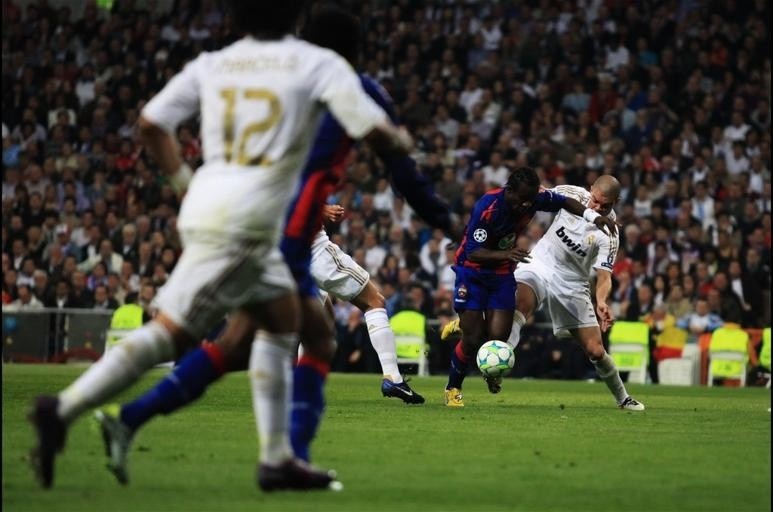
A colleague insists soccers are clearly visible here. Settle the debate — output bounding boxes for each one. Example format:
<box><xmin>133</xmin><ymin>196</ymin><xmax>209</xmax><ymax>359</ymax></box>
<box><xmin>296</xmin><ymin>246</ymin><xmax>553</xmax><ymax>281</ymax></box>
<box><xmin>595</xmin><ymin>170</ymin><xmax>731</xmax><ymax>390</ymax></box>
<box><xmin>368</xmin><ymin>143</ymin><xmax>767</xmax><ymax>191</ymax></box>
<box><xmin>476</xmin><ymin>340</ymin><xmax>517</xmax><ymax>379</ymax></box>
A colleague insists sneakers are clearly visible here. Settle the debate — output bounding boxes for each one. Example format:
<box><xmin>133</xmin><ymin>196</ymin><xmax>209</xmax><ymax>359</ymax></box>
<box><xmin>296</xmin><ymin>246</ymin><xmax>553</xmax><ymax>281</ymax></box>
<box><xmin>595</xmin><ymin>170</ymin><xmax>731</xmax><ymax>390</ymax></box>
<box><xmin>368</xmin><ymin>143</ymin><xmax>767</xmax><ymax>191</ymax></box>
<box><xmin>444</xmin><ymin>383</ymin><xmax>464</xmax><ymax>407</ymax></box>
<box><xmin>621</xmin><ymin>396</ymin><xmax>645</xmax><ymax>411</ymax></box>
<box><xmin>441</xmin><ymin>318</ymin><xmax>461</xmax><ymax>340</ymax></box>
<box><xmin>256</xmin><ymin>458</ymin><xmax>343</xmax><ymax>492</ymax></box>
<box><xmin>28</xmin><ymin>394</ymin><xmax>66</xmax><ymax>490</ymax></box>
<box><xmin>94</xmin><ymin>402</ymin><xmax>132</xmax><ymax>486</ymax></box>
<box><xmin>483</xmin><ymin>374</ymin><xmax>502</xmax><ymax>394</ymax></box>
<box><xmin>382</xmin><ymin>379</ymin><xmax>426</xmax><ymax>405</ymax></box>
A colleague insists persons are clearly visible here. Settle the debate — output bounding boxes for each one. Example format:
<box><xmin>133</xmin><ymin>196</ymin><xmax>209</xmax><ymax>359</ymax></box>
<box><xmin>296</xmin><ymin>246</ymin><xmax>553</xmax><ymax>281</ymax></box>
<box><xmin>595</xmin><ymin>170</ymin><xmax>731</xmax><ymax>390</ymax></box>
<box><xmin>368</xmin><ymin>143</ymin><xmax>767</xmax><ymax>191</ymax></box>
<box><xmin>27</xmin><ymin>0</ymin><xmax>401</xmax><ymax>489</ymax></box>
<box><xmin>97</xmin><ymin>11</ymin><xmax>466</xmax><ymax>488</ymax></box>
